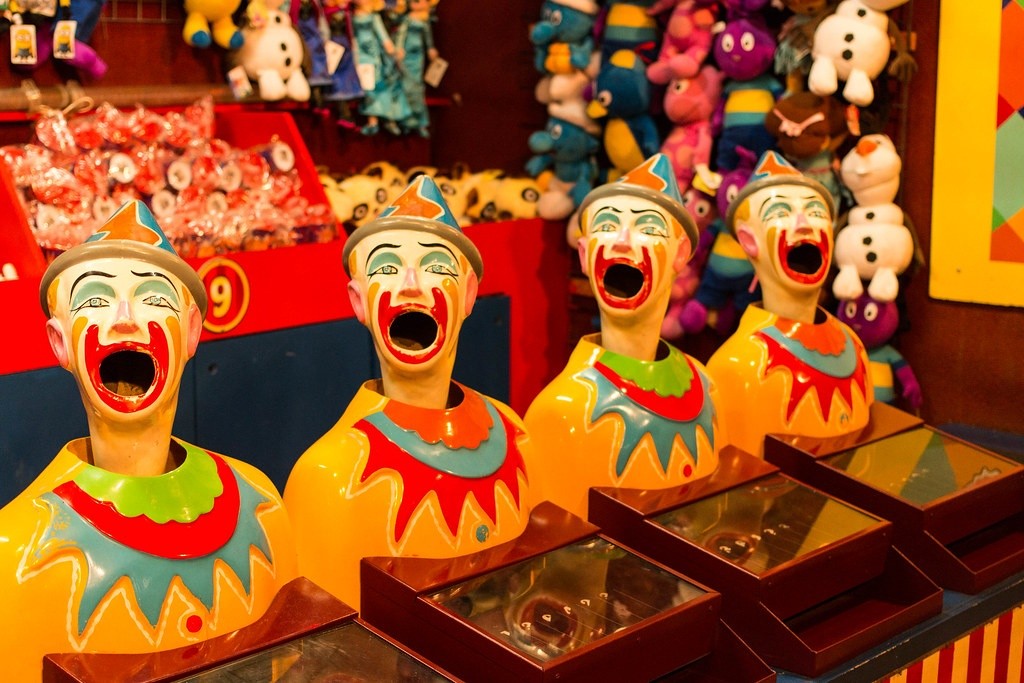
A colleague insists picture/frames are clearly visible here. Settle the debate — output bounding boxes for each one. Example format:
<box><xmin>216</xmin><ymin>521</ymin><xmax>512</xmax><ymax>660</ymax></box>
<box><xmin>929</xmin><ymin>1</ymin><xmax>1024</xmax><ymax>309</ymax></box>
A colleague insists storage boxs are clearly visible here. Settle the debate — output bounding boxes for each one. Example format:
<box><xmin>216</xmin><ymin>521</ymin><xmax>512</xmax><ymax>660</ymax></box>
<box><xmin>41</xmin><ymin>401</ymin><xmax>1024</xmax><ymax>683</ymax></box>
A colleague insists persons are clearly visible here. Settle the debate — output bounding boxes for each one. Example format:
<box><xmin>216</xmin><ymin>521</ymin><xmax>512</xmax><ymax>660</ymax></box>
<box><xmin>521</xmin><ymin>153</ymin><xmax>727</xmax><ymax>523</ymax></box>
<box><xmin>0</xmin><ymin>202</ymin><xmax>295</xmax><ymax>683</ymax></box>
<box><xmin>708</xmin><ymin>151</ymin><xmax>875</xmax><ymax>468</ymax></box>
<box><xmin>284</xmin><ymin>175</ymin><xmax>539</xmax><ymax>617</ymax></box>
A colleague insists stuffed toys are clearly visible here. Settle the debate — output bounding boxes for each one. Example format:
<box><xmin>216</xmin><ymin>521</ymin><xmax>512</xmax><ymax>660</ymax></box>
<box><xmin>0</xmin><ymin>0</ymin><xmax>108</xmax><ymax>77</ymax></box>
<box><xmin>182</xmin><ymin>0</ymin><xmax>925</xmax><ymax>403</ymax></box>
